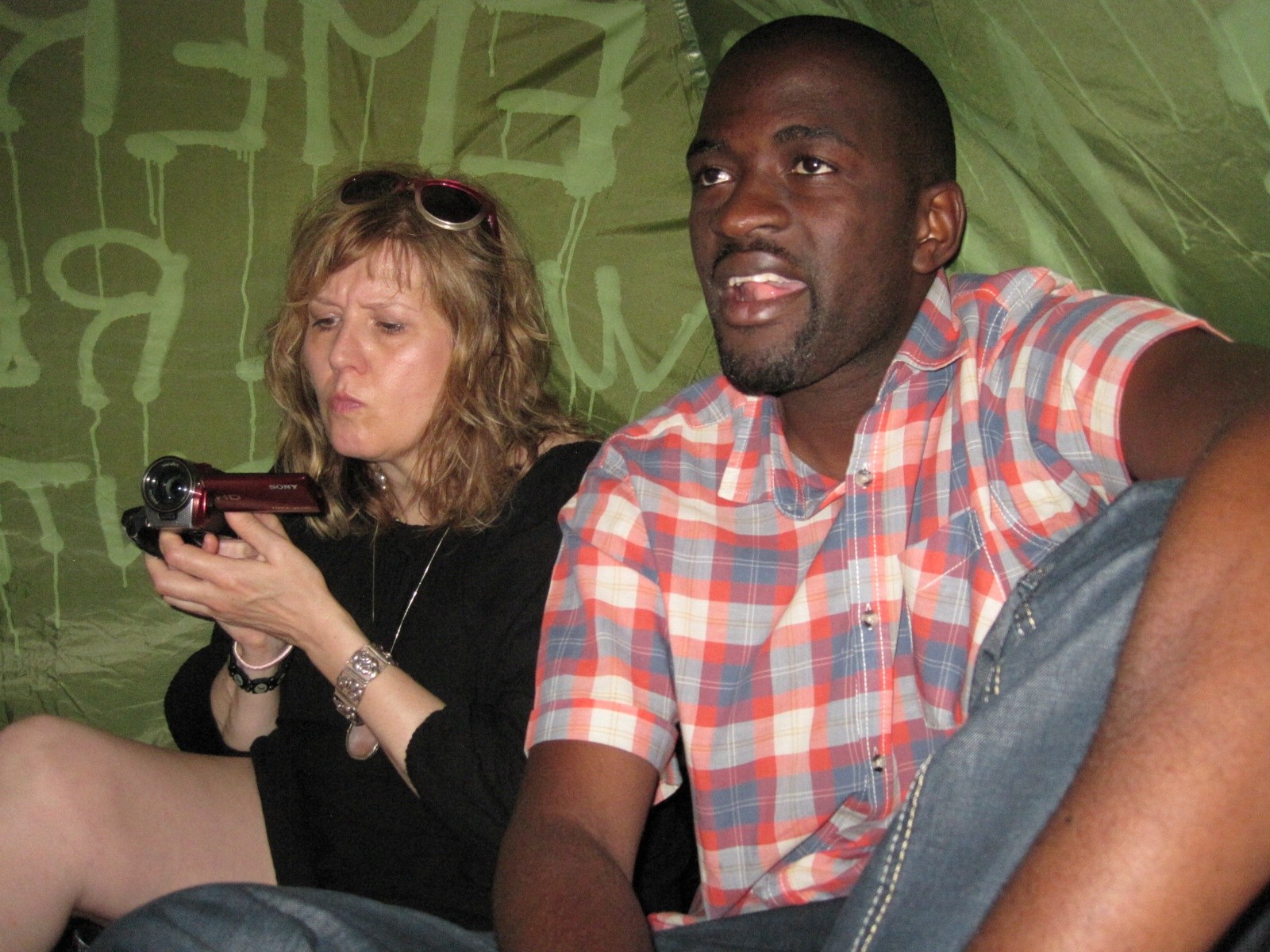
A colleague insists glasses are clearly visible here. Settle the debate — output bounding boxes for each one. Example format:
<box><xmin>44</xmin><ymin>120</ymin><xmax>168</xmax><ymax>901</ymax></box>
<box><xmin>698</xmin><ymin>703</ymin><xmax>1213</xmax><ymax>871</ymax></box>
<box><xmin>337</xmin><ymin>169</ymin><xmax>501</xmax><ymax>247</ymax></box>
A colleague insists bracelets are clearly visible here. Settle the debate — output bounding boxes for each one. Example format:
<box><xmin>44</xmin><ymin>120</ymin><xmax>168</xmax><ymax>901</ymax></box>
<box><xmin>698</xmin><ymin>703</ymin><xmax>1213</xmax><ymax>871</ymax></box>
<box><xmin>231</xmin><ymin>637</ymin><xmax>293</xmax><ymax>670</ymax></box>
<box><xmin>226</xmin><ymin>651</ymin><xmax>286</xmax><ymax>694</ymax></box>
<box><xmin>334</xmin><ymin>644</ymin><xmax>396</xmax><ymax>726</ymax></box>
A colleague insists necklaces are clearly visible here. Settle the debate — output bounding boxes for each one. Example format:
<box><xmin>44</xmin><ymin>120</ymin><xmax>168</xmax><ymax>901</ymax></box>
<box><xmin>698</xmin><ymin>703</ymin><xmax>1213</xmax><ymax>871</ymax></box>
<box><xmin>378</xmin><ymin>466</ymin><xmax>402</xmax><ymax>525</ymax></box>
<box><xmin>342</xmin><ymin>510</ymin><xmax>466</xmax><ymax>761</ymax></box>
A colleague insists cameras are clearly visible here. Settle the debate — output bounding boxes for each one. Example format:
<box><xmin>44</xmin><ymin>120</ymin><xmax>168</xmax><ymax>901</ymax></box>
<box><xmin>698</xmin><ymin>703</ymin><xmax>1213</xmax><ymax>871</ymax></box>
<box><xmin>120</xmin><ymin>454</ymin><xmax>327</xmax><ymax>563</ymax></box>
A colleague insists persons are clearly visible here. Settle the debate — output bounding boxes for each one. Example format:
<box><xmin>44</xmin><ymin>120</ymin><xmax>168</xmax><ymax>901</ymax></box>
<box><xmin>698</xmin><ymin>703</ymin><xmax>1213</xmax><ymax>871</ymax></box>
<box><xmin>0</xmin><ymin>165</ymin><xmax>699</xmax><ymax>952</ymax></box>
<box><xmin>96</xmin><ymin>11</ymin><xmax>1269</xmax><ymax>952</ymax></box>
